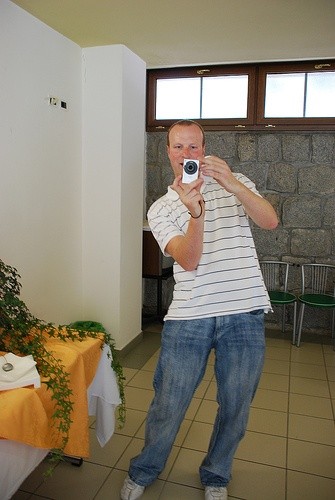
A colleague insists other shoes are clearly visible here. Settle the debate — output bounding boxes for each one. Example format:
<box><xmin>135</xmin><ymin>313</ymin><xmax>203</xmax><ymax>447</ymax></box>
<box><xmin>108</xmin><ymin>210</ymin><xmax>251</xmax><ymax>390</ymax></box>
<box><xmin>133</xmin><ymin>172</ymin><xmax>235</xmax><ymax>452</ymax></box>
<box><xmin>120</xmin><ymin>474</ymin><xmax>145</xmax><ymax>499</ymax></box>
<box><xmin>203</xmin><ymin>485</ymin><xmax>227</xmax><ymax>500</ymax></box>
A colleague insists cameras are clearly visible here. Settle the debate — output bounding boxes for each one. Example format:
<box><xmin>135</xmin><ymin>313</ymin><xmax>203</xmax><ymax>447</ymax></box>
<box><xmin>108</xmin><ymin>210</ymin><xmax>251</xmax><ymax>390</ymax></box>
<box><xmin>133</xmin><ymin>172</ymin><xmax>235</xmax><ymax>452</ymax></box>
<box><xmin>182</xmin><ymin>159</ymin><xmax>200</xmax><ymax>183</ymax></box>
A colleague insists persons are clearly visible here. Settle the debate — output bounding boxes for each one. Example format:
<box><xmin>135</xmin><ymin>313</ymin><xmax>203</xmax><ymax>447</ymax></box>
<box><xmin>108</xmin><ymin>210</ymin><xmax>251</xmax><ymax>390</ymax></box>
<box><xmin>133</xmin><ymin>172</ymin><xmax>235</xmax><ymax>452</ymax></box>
<box><xmin>120</xmin><ymin>119</ymin><xmax>279</xmax><ymax>500</ymax></box>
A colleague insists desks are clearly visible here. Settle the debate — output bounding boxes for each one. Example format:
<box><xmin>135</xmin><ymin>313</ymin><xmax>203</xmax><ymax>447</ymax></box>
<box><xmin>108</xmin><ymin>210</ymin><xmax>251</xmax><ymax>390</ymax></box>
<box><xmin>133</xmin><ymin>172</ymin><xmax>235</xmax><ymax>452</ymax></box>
<box><xmin>0</xmin><ymin>327</ymin><xmax>122</xmax><ymax>500</ymax></box>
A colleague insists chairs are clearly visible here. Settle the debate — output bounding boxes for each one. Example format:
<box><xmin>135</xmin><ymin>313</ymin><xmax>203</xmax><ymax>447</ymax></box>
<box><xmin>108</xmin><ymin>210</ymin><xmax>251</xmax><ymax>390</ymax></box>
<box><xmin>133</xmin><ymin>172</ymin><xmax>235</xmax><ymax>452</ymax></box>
<box><xmin>297</xmin><ymin>263</ymin><xmax>335</xmax><ymax>348</ymax></box>
<box><xmin>259</xmin><ymin>260</ymin><xmax>298</xmax><ymax>345</ymax></box>
<box><xmin>142</xmin><ymin>226</ymin><xmax>173</xmax><ymax>320</ymax></box>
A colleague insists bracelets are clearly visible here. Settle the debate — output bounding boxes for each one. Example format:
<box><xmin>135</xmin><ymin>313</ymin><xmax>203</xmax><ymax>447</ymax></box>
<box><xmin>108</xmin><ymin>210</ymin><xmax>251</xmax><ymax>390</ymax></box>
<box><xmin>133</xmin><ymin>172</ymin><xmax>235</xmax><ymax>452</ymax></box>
<box><xmin>187</xmin><ymin>198</ymin><xmax>205</xmax><ymax>218</ymax></box>
